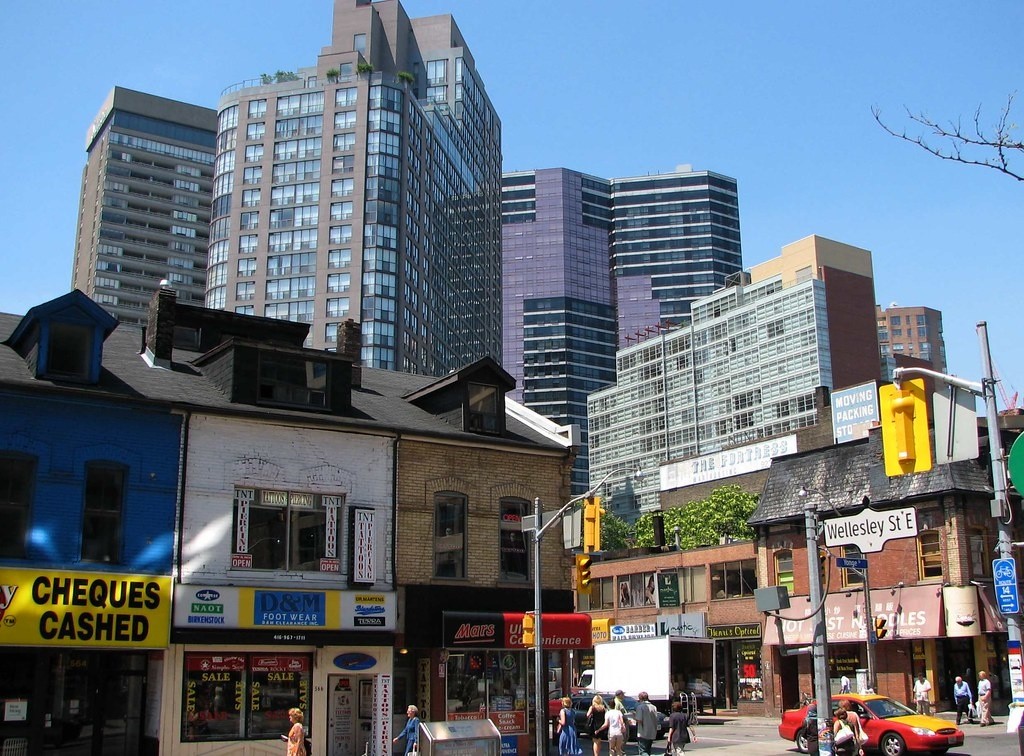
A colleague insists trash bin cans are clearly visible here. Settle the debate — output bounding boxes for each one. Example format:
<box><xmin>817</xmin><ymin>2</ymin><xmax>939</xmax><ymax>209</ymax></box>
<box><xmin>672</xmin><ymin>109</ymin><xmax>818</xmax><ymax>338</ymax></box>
<box><xmin>420</xmin><ymin>719</ymin><xmax>502</xmax><ymax>756</ymax></box>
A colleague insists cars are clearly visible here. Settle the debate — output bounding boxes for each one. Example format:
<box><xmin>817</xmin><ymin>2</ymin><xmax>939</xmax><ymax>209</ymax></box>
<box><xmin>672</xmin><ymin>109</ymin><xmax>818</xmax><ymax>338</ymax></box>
<box><xmin>571</xmin><ymin>695</ymin><xmax>670</xmax><ymax>742</ymax></box>
<box><xmin>779</xmin><ymin>694</ymin><xmax>965</xmax><ymax>756</ymax></box>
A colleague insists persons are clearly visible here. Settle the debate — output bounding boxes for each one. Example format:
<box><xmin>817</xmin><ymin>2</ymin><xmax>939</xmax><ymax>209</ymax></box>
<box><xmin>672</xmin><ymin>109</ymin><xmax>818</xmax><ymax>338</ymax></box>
<box><xmin>912</xmin><ymin>672</ymin><xmax>932</xmax><ymax>715</ymax></box>
<box><xmin>645</xmin><ymin>575</ymin><xmax>656</xmax><ymax>605</ymax></box>
<box><xmin>556</xmin><ymin>697</ymin><xmax>585</xmax><ymax>756</ymax></box>
<box><xmin>801</xmin><ymin>700</ymin><xmax>864</xmax><ymax>756</ymax></box>
<box><xmin>620</xmin><ymin>583</ymin><xmax>630</xmax><ymax>607</ymax></box>
<box><xmin>282</xmin><ymin>708</ymin><xmax>306</xmax><ymax>756</ymax></box>
<box><xmin>840</xmin><ymin>672</ymin><xmax>851</xmax><ymax>694</ymax></box>
<box><xmin>977</xmin><ymin>671</ymin><xmax>994</xmax><ymax>726</ymax></box>
<box><xmin>633</xmin><ymin>692</ymin><xmax>657</xmax><ymax>756</ymax></box>
<box><xmin>586</xmin><ymin>689</ymin><xmax>633</xmax><ymax>756</ymax></box>
<box><xmin>393</xmin><ymin>704</ymin><xmax>421</xmax><ymax>756</ymax></box>
<box><xmin>667</xmin><ymin>702</ymin><xmax>698</xmax><ymax>756</ymax></box>
<box><xmin>953</xmin><ymin>676</ymin><xmax>974</xmax><ymax>725</ymax></box>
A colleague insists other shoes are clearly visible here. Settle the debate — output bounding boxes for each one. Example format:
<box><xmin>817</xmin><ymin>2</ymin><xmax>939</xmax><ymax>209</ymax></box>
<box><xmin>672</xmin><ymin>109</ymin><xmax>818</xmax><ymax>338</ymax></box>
<box><xmin>988</xmin><ymin>721</ymin><xmax>994</xmax><ymax>725</ymax></box>
<box><xmin>980</xmin><ymin>723</ymin><xmax>985</xmax><ymax>727</ymax></box>
<box><xmin>966</xmin><ymin>719</ymin><xmax>972</xmax><ymax>723</ymax></box>
<box><xmin>956</xmin><ymin>722</ymin><xmax>960</xmax><ymax>725</ymax></box>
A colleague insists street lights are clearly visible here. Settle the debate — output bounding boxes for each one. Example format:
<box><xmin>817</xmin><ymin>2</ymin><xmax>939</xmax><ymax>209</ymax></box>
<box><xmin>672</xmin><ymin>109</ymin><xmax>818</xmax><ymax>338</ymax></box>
<box><xmin>534</xmin><ymin>469</ymin><xmax>644</xmax><ymax>756</ymax></box>
<box><xmin>799</xmin><ymin>486</ymin><xmax>877</xmax><ymax>694</ymax></box>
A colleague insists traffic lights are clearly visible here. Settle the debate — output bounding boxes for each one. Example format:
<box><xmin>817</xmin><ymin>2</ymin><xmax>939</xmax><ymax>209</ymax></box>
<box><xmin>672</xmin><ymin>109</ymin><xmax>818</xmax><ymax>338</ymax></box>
<box><xmin>576</xmin><ymin>553</ymin><xmax>592</xmax><ymax>594</ymax></box>
<box><xmin>875</xmin><ymin>617</ymin><xmax>887</xmax><ymax>638</ymax></box>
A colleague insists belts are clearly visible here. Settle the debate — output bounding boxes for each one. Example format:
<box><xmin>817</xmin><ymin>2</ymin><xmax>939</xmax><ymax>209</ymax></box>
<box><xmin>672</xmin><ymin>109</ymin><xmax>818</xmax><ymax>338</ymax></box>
<box><xmin>980</xmin><ymin>693</ymin><xmax>985</xmax><ymax>696</ymax></box>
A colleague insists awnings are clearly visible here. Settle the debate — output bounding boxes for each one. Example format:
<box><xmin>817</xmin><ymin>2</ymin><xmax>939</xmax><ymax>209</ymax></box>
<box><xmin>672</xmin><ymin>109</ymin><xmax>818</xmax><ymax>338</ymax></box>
<box><xmin>978</xmin><ymin>587</ymin><xmax>1024</xmax><ymax>633</ymax></box>
<box><xmin>440</xmin><ymin>611</ymin><xmax>592</xmax><ymax>649</ymax></box>
<box><xmin>763</xmin><ymin>584</ymin><xmax>946</xmax><ymax>646</ymax></box>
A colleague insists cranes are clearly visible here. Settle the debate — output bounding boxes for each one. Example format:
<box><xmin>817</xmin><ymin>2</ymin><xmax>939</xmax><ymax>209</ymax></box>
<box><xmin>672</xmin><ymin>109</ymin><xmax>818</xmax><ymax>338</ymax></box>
<box><xmin>975</xmin><ymin>330</ymin><xmax>1018</xmax><ymax>409</ymax></box>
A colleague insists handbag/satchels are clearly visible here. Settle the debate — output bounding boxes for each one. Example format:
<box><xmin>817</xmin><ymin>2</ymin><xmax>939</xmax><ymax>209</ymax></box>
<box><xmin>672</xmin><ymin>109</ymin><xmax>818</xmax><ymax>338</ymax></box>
<box><xmin>968</xmin><ymin>704</ymin><xmax>977</xmax><ymax>718</ymax></box>
<box><xmin>586</xmin><ymin>717</ymin><xmax>593</xmax><ymax>733</ymax></box>
<box><xmin>304</xmin><ymin>739</ymin><xmax>312</xmax><ymax>756</ymax></box>
<box><xmin>664</xmin><ymin>742</ymin><xmax>673</xmax><ymax>756</ymax></box>
<box><xmin>833</xmin><ymin>720</ymin><xmax>854</xmax><ymax>745</ymax></box>
<box><xmin>857</xmin><ymin>729</ymin><xmax>868</xmax><ymax>744</ymax></box>
<box><xmin>975</xmin><ymin>701</ymin><xmax>982</xmax><ymax>718</ymax></box>
<box><xmin>408</xmin><ymin>746</ymin><xmax>417</xmax><ymax>756</ymax></box>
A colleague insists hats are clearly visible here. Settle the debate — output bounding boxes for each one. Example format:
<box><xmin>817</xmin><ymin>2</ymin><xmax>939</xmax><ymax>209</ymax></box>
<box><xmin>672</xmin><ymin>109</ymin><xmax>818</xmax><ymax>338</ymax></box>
<box><xmin>615</xmin><ymin>690</ymin><xmax>626</xmax><ymax>695</ymax></box>
<box><xmin>809</xmin><ymin>704</ymin><xmax>816</xmax><ymax>711</ymax></box>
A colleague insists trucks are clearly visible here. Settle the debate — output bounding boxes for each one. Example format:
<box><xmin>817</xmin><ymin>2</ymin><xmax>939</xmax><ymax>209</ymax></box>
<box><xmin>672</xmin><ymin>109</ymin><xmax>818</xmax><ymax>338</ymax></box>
<box><xmin>577</xmin><ymin>635</ymin><xmax>717</xmax><ymax>712</ymax></box>
